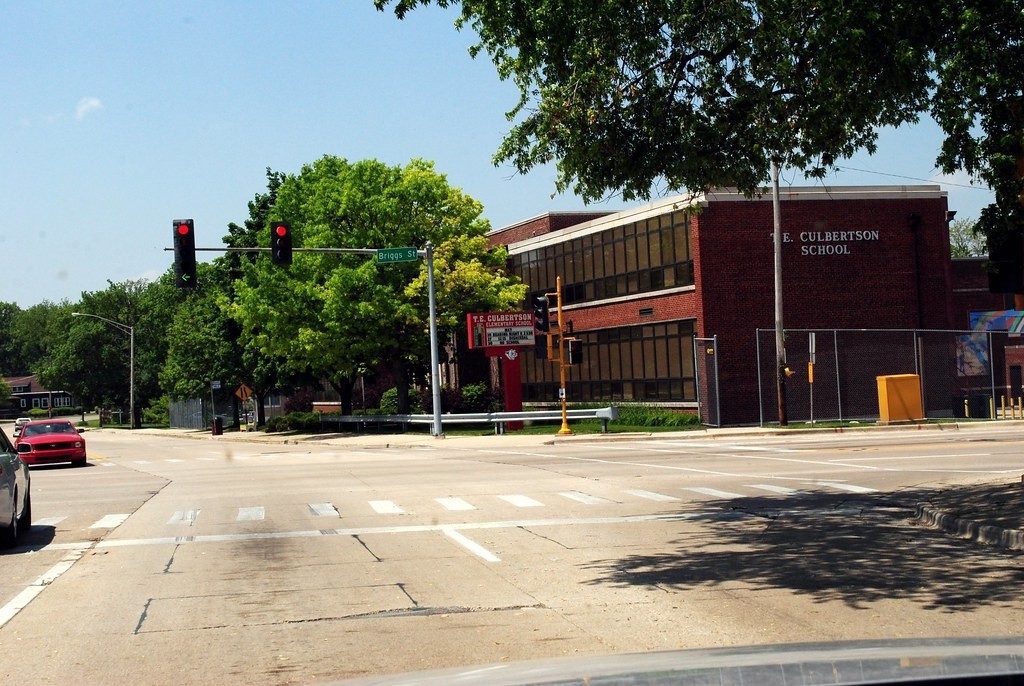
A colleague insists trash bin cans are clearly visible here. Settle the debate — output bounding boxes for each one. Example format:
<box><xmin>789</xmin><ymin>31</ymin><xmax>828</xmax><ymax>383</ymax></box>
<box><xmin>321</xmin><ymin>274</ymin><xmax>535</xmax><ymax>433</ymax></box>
<box><xmin>249</xmin><ymin>416</ymin><xmax>253</xmax><ymax>423</ymax></box>
<box><xmin>212</xmin><ymin>417</ymin><xmax>223</xmax><ymax>435</ymax></box>
<box><xmin>242</xmin><ymin>414</ymin><xmax>250</xmax><ymax>424</ymax></box>
<box><xmin>969</xmin><ymin>394</ymin><xmax>990</xmax><ymax>418</ymax></box>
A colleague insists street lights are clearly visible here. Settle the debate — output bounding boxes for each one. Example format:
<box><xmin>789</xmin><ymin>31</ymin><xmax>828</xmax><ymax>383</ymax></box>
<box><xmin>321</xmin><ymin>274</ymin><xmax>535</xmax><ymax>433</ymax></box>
<box><xmin>71</xmin><ymin>310</ymin><xmax>135</xmax><ymax>429</ymax></box>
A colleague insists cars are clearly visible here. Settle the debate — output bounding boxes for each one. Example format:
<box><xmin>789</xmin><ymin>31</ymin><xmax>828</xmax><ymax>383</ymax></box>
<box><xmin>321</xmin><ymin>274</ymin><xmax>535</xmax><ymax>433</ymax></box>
<box><xmin>0</xmin><ymin>426</ymin><xmax>32</xmax><ymax>547</ymax></box>
<box><xmin>16</xmin><ymin>417</ymin><xmax>32</xmax><ymax>432</ymax></box>
<box><xmin>15</xmin><ymin>418</ymin><xmax>87</xmax><ymax>471</ymax></box>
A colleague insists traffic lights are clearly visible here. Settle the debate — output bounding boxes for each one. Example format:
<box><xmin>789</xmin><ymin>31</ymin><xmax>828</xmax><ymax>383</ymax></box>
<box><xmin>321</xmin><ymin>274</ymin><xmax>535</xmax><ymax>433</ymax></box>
<box><xmin>531</xmin><ymin>294</ymin><xmax>548</xmax><ymax>333</ymax></box>
<box><xmin>174</xmin><ymin>219</ymin><xmax>196</xmax><ymax>290</ymax></box>
<box><xmin>569</xmin><ymin>339</ymin><xmax>583</xmax><ymax>365</ymax></box>
<box><xmin>271</xmin><ymin>221</ymin><xmax>293</xmax><ymax>267</ymax></box>
